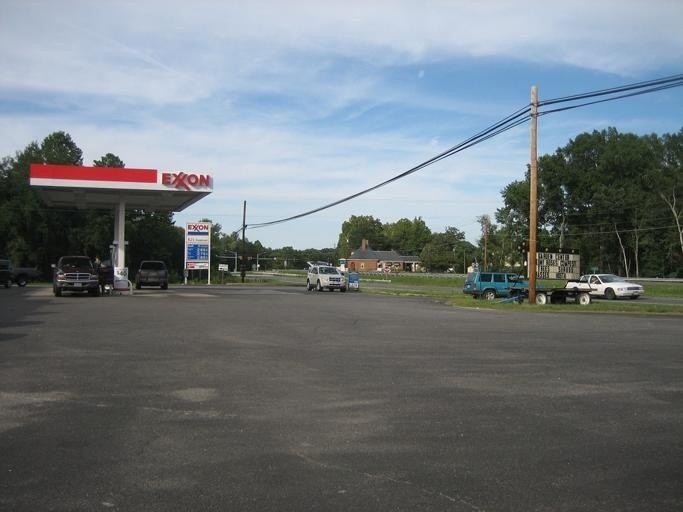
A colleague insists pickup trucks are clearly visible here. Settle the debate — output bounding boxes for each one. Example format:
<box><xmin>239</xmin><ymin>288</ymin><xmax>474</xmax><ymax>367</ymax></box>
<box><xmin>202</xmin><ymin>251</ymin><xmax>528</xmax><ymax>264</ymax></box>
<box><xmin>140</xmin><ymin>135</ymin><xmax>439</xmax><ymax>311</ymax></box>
<box><xmin>0</xmin><ymin>259</ymin><xmax>36</xmax><ymax>288</ymax></box>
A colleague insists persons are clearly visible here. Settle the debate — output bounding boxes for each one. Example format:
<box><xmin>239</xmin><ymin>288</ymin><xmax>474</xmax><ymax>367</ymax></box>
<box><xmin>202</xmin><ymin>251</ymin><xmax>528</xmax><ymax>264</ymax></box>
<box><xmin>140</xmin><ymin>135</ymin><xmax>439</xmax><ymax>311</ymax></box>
<box><xmin>95</xmin><ymin>255</ymin><xmax>105</xmax><ymax>296</ymax></box>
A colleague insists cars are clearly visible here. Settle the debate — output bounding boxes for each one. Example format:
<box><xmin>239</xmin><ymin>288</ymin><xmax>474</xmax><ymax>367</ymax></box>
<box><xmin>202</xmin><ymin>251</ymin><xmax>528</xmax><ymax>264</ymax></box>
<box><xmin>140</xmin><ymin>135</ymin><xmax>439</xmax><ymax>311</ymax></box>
<box><xmin>135</xmin><ymin>260</ymin><xmax>168</xmax><ymax>289</ymax></box>
<box><xmin>564</xmin><ymin>273</ymin><xmax>644</xmax><ymax>300</ymax></box>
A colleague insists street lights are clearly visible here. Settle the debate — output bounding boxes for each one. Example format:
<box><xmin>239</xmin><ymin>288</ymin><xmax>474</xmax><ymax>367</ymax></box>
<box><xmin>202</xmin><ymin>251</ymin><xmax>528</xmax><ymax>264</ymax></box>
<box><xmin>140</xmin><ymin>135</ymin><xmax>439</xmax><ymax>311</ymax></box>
<box><xmin>256</xmin><ymin>252</ymin><xmax>267</xmax><ymax>272</ymax></box>
<box><xmin>224</xmin><ymin>249</ymin><xmax>237</xmax><ymax>272</ymax></box>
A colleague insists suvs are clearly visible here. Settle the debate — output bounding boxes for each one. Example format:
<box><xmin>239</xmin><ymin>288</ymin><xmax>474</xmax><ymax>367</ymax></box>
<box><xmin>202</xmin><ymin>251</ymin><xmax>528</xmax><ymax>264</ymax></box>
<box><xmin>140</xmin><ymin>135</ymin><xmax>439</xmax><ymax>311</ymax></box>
<box><xmin>50</xmin><ymin>255</ymin><xmax>113</xmax><ymax>297</ymax></box>
<box><xmin>305</xmin><ymin>260</ymin><xmax>346</xmax><ymax>292</ymax></box>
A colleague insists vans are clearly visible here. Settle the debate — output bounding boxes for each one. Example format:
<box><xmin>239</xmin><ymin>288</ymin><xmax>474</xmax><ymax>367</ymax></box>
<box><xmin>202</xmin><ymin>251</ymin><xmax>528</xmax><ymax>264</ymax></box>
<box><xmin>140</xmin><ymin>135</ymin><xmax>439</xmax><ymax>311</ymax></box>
<box><xmin>462</xmin><ymin>272</ymin><xmax>528</xmax><ymax>300</ymax></box>
